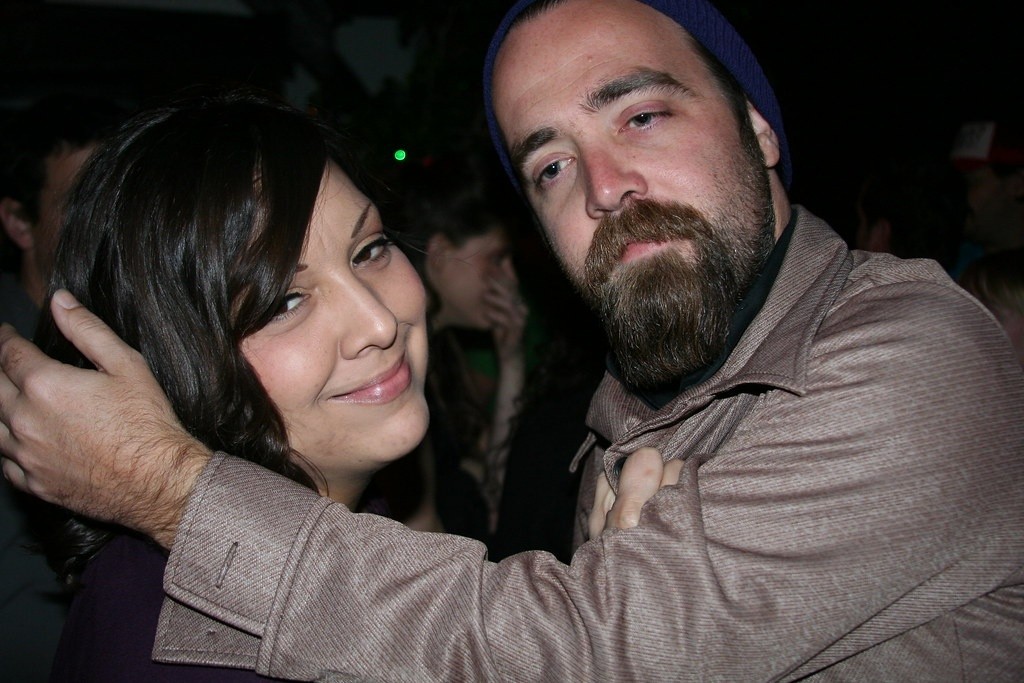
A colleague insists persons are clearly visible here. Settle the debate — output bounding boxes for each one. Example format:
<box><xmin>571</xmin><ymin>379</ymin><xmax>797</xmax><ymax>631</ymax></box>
<box><xmin>1</xmin><ymin>0</ymin><xmax>1024</xmax><ymax>683</ymax></box>
<box><xmin>1</xmin><ymin>113</ymin><xmax>1022</xmax><ymax>681</ymax></box>
<box><xmin>7</xmin><ymin>90</ymin><xmax>431</xmax><ymax>681</ymax></box>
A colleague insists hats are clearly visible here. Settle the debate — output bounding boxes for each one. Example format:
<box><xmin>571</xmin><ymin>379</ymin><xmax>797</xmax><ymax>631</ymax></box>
<box><xmin>482</xmin><ymin>0</ymin><xmax>789</xmax><ymax>225</ymax></box>
